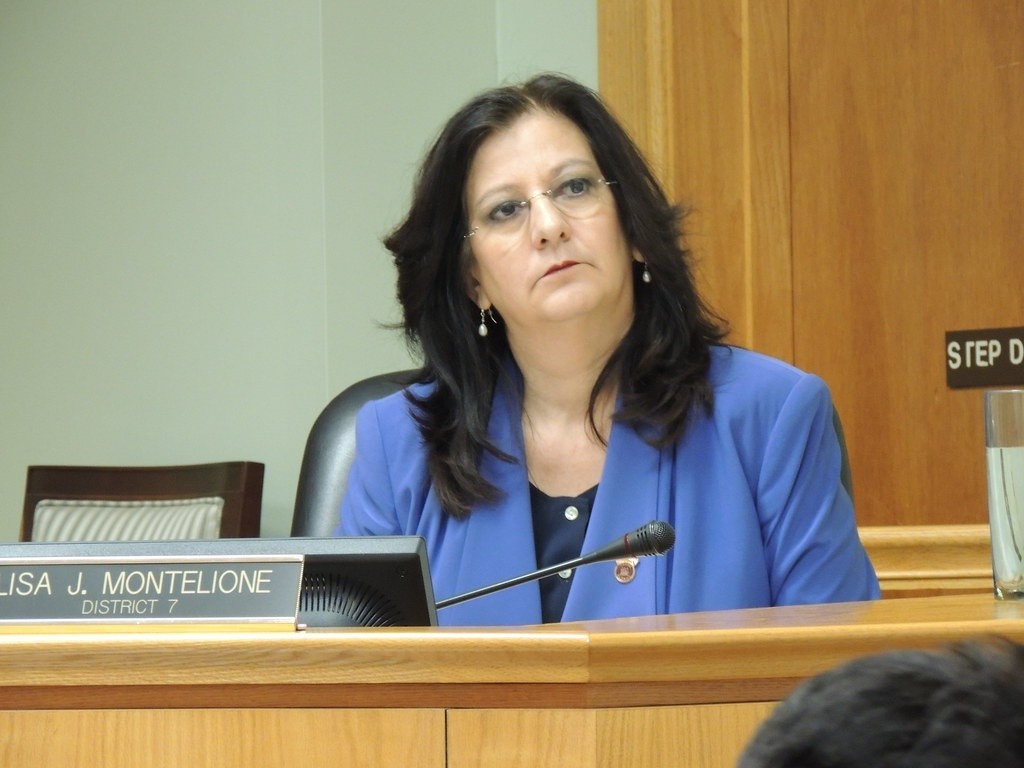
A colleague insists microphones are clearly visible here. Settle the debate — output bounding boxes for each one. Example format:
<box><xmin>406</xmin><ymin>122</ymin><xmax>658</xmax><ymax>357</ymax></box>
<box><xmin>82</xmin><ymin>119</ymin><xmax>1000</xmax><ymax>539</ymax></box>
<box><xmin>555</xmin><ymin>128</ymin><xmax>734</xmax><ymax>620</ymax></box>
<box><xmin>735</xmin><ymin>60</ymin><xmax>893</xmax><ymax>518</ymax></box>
<box><xmin>435</xmin><ymin>521</ymin><xmax>676</xmax><ymax>609</ymax></box>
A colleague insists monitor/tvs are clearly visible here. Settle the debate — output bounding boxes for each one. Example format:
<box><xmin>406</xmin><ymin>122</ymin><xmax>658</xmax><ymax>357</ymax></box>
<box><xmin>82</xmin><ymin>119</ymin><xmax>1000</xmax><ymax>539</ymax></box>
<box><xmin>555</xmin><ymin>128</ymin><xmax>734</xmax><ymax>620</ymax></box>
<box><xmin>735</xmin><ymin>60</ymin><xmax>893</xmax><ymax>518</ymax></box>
<box><xmin>0</xmin><ymin>536</ymin><xmax>439</xmax><ymax>627</ymax></box>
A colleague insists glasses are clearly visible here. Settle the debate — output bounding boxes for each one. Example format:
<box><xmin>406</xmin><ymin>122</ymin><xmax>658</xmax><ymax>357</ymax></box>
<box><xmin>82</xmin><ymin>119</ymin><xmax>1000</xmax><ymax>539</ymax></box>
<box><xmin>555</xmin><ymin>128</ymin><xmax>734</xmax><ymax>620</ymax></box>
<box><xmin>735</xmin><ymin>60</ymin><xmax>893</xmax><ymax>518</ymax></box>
<box><xmin>462</xmin><ymin>171</ymin><xmax>617</xmax><ymax>239</ymax></box>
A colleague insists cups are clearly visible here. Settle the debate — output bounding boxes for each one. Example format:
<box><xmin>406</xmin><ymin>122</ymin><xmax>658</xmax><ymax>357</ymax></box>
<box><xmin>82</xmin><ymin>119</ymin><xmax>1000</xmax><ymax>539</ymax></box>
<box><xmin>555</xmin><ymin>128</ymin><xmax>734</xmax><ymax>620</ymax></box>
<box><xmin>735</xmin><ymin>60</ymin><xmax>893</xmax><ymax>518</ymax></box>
<box><xmin>983</xmin><ymin>388</ymin><xmax>1024</xmax><ymax>602</ymax></box>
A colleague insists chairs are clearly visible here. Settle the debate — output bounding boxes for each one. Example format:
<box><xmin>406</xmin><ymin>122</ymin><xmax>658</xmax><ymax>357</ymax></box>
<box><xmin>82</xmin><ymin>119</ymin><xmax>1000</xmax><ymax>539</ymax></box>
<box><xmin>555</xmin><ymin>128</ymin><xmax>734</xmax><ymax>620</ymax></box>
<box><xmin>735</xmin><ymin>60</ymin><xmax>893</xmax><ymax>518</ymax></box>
<box><xmin>288</xmin><ymin>366</ymin><xmax>424</xmax><ymax>534</ymax></box>
<box><xmin>18</xmin><ymin>461</ymin><xmax>265</xmax><ymax>541</ymax></box>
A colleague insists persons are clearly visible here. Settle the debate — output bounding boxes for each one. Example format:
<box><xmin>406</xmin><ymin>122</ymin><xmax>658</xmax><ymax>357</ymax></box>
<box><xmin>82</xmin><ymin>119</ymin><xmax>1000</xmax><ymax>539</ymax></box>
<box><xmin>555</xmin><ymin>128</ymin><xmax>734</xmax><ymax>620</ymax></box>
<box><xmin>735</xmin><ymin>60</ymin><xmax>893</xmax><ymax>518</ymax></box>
<box><xmin>735</xmin><ymin>638</ymin><xmax>1024</xmax><ymax>768</ymax></box>
<box><xmin>328</xmin><ymin>72</ymin><xmax>882</xmax><ymax>628</ymax></box>
